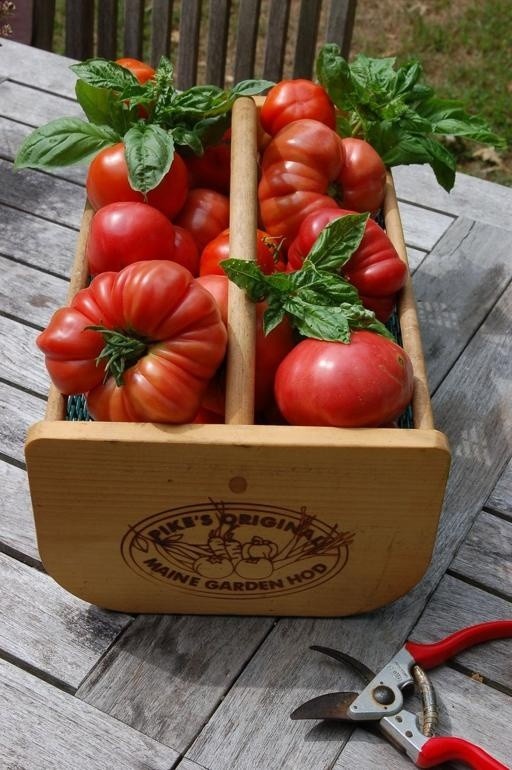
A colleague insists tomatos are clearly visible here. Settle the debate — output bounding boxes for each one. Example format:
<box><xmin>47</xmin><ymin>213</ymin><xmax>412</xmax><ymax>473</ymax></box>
<box><xmin>35</xmin><ymin>57</ymin><xmax>415</xmax><ymax>428</ymax></box>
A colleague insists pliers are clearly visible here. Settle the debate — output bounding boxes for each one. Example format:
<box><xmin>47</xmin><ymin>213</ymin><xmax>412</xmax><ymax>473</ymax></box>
<box><xmin>291</xmin><ymin>620</ymin><xmax>512</xmax><ymax>770</ymax></box>
